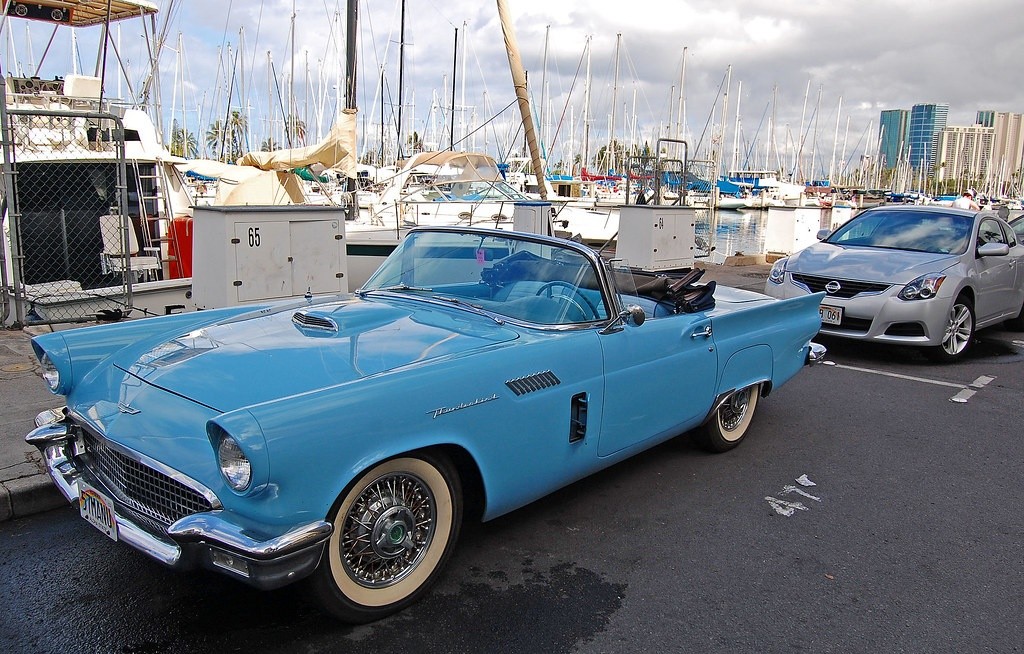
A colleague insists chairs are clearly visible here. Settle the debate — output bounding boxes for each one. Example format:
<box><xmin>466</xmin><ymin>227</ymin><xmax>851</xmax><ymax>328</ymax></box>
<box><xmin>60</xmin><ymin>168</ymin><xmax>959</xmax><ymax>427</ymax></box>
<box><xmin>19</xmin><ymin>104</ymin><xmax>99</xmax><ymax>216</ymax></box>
<box><xmin>99</xmin><ymin>214</ymin><xmax>159</xmax><ymax>284</ymax></box>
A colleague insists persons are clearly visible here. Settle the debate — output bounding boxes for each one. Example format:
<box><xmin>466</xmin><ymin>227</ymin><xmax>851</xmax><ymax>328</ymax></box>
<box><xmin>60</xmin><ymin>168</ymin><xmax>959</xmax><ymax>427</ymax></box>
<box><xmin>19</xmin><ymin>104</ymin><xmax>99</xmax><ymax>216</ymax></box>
<box><xmin>950</xmin><ymin>192</ymin><xmax>980</xmax><ymax>211</ymax></box>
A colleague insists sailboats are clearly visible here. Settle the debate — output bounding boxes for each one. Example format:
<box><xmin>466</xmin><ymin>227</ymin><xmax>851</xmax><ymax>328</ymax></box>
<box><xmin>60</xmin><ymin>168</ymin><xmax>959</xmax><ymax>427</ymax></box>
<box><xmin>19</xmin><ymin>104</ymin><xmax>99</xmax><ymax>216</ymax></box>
<box><xmin>0</xmin><ymin>0</ymin><xmax>1024</xmax><ymax>332</ymax></box>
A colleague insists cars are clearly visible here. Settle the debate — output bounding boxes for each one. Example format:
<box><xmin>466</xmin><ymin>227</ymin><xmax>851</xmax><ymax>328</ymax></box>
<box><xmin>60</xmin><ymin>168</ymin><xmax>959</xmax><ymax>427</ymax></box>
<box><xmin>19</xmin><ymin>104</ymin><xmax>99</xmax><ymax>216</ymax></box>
<box><xmin>765</xmin><ymin>205</ymin><xmax>1024</xmax><ymax>365</ymax></box>
<box><xmin>1007</xmin><ymin>215</ymin><xmax>1024</xmax><ymax>246</ymax></box>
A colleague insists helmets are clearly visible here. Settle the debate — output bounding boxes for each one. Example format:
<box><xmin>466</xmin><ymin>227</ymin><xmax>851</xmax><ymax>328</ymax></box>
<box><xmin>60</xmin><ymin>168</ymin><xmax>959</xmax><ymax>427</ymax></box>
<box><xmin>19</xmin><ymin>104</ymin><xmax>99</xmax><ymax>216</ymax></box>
<box><xmin>963</xmin><ymin>189</ymin><xmax>974</xmax><ymax>196</ymax></box>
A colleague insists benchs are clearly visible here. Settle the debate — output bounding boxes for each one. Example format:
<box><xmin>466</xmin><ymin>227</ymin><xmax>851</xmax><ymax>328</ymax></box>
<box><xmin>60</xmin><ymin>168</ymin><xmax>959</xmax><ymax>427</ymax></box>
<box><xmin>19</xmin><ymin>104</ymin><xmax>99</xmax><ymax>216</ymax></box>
<box><xmin>492</xmin><ymin>280</ymin><xmax>670</xmax><ymax>320</ymax></box>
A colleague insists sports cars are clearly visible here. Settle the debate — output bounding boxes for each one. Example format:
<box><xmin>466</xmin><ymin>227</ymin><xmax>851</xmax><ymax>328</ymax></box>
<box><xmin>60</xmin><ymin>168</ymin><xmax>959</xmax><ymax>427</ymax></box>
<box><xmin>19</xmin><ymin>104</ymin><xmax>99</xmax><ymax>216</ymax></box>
<box><xmin>25</xmin><ymin>225</ymin><xmax>829</xmax><ymax>626</ymax></box>
